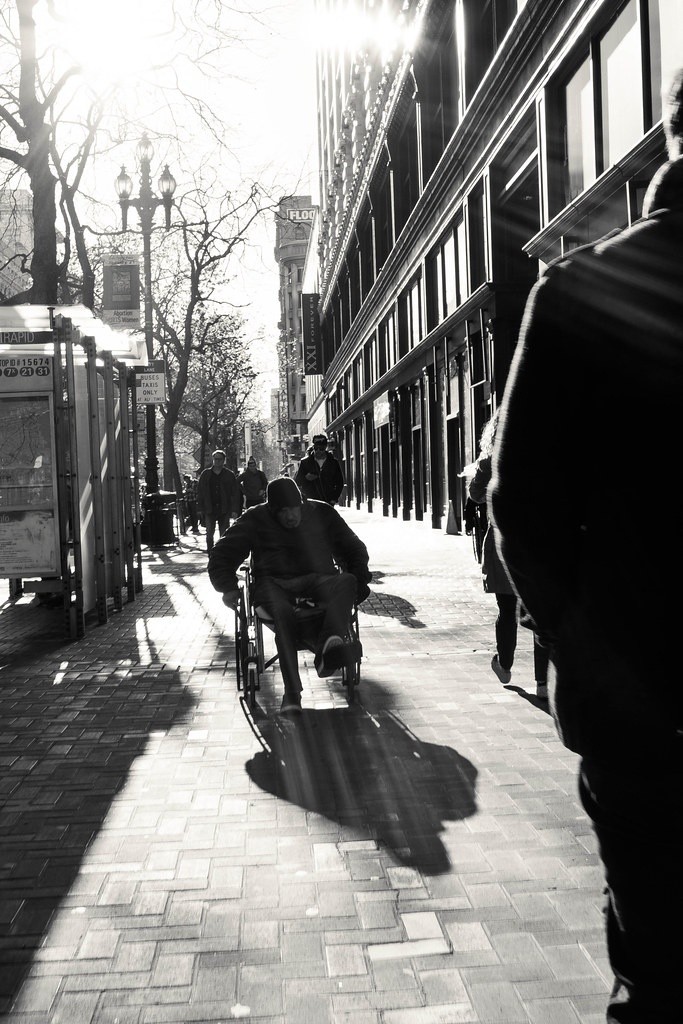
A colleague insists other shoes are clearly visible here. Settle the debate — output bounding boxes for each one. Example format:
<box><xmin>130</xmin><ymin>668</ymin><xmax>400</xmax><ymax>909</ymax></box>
<box><xmin>491</xmin><ymin>654</ymin><xmax>512</xmax><ymax>684</ymax></box>
<box><xmin>280</xmin><ymin>693</ymin><xmax>303</xmax><ymax>713</ymax></box>
<box><xmin>317</xmin><ymin>636</ymin><xmax>343</xmax><ymax>678</ymax></box>
<box><xmin>537</xmin><ymin>681</ymin><xmax>548</xmax><ymax>697</ymax></box>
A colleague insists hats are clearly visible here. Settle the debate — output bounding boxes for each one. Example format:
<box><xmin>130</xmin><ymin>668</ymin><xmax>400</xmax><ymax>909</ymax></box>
<box><xmin>267</xmin><ymin>477</ymin><xmax>302</xmax><ymax>514</ymax></box>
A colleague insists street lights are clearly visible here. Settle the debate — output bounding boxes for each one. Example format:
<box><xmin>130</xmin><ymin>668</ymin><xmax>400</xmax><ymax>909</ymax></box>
<box><xmin>113</xmin><ymin>131</ymin><xmax>177</xmax><ymax>549</ymax></box>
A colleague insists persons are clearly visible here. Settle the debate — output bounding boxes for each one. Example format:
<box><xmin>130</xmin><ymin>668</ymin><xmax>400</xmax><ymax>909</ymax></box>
<box><xmin>459</xmin><ymin>68</ymin><xmax>683</xmax><ymax>1024</ymax></box>
<box><xmin>184</xmin><ymin>450</ymin><xmax>267</xmax><ymax>558</ymax></box>
<box><xmin>207</xmin><ymin>478</ymin><xmax>372</xmax><ymax>715</ymax></box>
<box><xmin>295</xmin><ymin>434</ymin><xmax>344</xmax><ymax>508</ymax></box>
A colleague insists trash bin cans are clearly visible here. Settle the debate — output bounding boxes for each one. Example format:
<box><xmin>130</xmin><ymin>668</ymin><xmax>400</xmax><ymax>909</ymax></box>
<box><xmin>146</xmin><ymin>490</ymin><xmax>177</xmax><ymax>546</ymax></box>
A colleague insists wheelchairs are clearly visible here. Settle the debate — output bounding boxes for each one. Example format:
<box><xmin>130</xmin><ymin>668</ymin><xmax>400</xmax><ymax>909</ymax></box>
<box><xmin>231</xmin><ymin>560</ymin><xmax>363</xmax><ymax>710</ymax></box>
